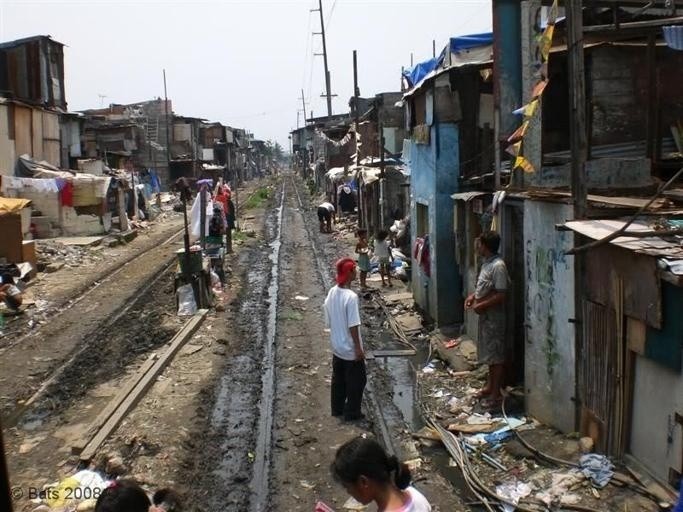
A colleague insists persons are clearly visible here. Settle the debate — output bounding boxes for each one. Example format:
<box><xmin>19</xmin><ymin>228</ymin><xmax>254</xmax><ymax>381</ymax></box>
<box><xmin>354</xmin><ymin>229</ymin><xmax>373</xmax><ymax>289</ymax></box>
<box><xmin>461</xmin><ymin>229</ymin><xmax>510</xmax><ymax>409</ymax></box>
<box><xmin>0</xmin><ymin>273</ymin><xmax>22</xmax><ymax>309</ymax></box>
<box><xmin>321</xmin><ymin>257</ymin><xmax>368</xmax><ymax>421</ymax></box>
<box><xmin>375</xmin><ymin>230</ymin><xmax>396</xmax><ymax>286</ymax></box>
<box><xmin>330</xmin><ymin>435</ymin><xmax>434</xmax><ymax>512</ymax></box>
<box><xmin>90</xmin><ymin>479</ymin><xmax>152</xmax><ymax>510</ymax></box>
<box><xmin>316</xmin><ymin>201</ymin><xmax>338</xmax><ymax>234</ymax></box>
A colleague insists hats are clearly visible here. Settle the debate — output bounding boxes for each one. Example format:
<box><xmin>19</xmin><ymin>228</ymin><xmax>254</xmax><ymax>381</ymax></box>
<box><xmin>336</xmin><ymin>257</ymin><xmax>357</xmax><ymax>272</ymax></box>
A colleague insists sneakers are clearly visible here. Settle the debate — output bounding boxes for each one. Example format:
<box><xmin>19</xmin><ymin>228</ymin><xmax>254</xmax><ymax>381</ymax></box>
<box><xmin>331</xmin><ymin>413</ymin><xmax>366</xmax><ymax>421</ymax></box>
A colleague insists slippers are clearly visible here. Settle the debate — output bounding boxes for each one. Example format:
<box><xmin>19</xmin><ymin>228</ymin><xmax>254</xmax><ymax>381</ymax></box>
<box><xmin>473</xmin><ymin>388</ymin><xmax>504</xmax><ymax>408</ymax></box>
